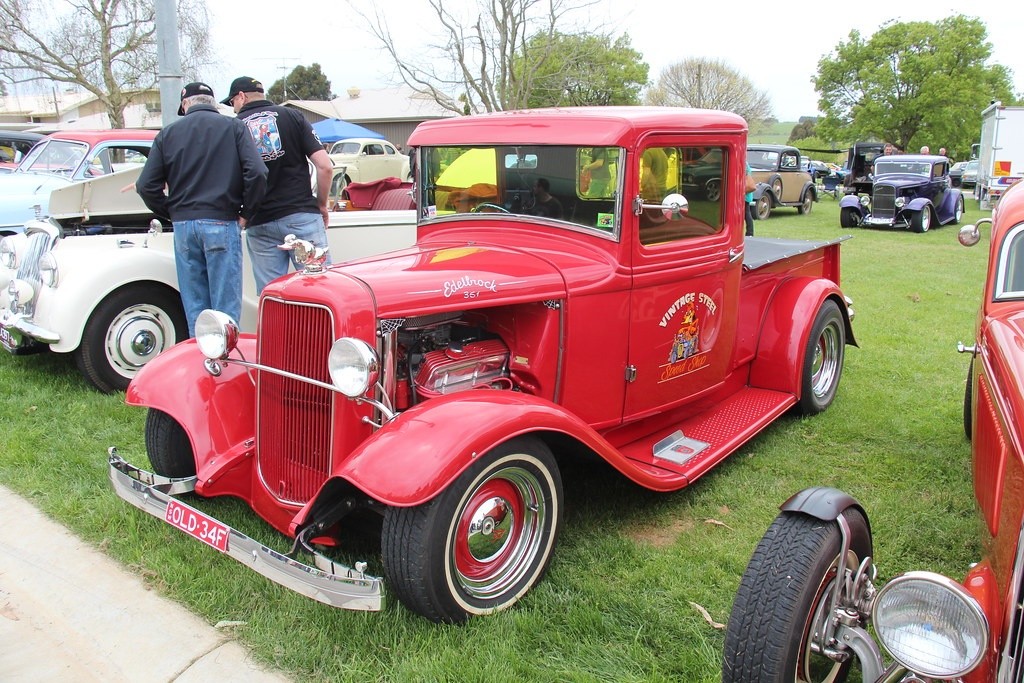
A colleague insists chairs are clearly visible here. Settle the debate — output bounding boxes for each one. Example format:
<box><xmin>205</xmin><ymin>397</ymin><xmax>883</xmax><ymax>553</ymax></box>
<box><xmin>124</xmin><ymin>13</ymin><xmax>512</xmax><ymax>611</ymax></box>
<box><xmin>817</xmin><ymin>169</ymin><xmax>841</xmax><ymax>202</ymax></box>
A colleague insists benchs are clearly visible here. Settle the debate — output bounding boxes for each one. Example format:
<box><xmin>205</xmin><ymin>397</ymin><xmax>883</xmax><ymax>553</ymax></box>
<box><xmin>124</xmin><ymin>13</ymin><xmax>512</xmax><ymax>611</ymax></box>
<box><xmin>372</xmin><ymin>188</ymin><xmax>416</xmax><ymax>210</ymax></box>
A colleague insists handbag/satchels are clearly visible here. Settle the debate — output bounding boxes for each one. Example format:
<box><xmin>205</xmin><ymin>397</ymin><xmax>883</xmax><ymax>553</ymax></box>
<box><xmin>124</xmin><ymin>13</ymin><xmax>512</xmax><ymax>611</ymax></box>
<box><xmin>579</xmin><ymin>169</ymin><xmax>590</xmax><ymax>193</ymax></box>
<box><xmin>745</xmin><ymin>174</ymin><xmax>756</xmax><ymax>194</ymax></box>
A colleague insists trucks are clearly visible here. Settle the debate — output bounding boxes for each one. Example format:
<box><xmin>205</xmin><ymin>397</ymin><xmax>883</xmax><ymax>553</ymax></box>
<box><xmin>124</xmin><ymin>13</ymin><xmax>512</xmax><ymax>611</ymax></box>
<box><xmin>973</xmin><ymin>99</ymin><xmax>1024</xmax><ymax>210</ymax></box>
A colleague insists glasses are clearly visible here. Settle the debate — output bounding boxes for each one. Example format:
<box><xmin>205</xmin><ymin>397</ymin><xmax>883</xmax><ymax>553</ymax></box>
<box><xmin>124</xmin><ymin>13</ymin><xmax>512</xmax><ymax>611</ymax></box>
<box><xmin>229</xmin><ymin>94</ymin><xmax>248</xmax><ymax>105</ymax></box>
<box><xmin>181</xmin><ymin>99</ymin><xmax>191</xmax><ymax>115</ymax></box>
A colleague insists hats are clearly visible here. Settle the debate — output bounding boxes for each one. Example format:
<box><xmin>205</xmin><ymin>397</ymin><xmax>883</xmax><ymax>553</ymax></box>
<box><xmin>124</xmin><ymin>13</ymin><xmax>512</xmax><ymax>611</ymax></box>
<box><xmin>178</xmin><ymin>82</ymin><xmax>214</xmax><ymax>116</ymax></box>
<box><xmin>220</xmin><ymin>76</ymin><xmax>264</xmax><ymax>106</ymax></box>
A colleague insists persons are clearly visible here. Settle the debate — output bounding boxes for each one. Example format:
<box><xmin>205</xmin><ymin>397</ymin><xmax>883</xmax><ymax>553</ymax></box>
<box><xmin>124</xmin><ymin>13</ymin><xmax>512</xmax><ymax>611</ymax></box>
<box><xmin>324</xmin><ymin>143</ymin><xmax>329</xmax><ymax>154</ymax></box>
<box><xmin>870</xmin><ymin>144</ymin><xmax>904</xmax><ymax>172</ymax></box>
<box><xmin>136</xmin><ymin>83</ymin><xmax>269</xmax><ymax>338</ymax></box>
<box><xmin>409</xmin><ymin>147</ymin><xmax>460</xmax><ymax>178</ymax></box>
<box><xmin>584</xmin><ymin>147</ymin><xmax>611</xmax><ymax>197</ymax></box>
<box><xmin>396</xmin><ymin>144</ymin><xmax>404</xmax><ymax>153</ymax></box>
<box><xmin>534</xmin><ymin>179</ymin><xmax>563</xmax><ymax>219</ymax></box>
<box><xmin>921</xmin><ymin>146</ymin><xmax>929</xmax><ymax>155</ymax></box>
<box><xmin>940</xmin><ymin>148</ymin><xmax>946</xmax><ymax>155</ymax></box>
<box><xmin>220</xmin><ymin>76</ymin><xmax>333</xmax><ymax>297</ymax></box>
<box><xmin>744</xmin><ymin>163</ymin><xmax>754</xmax><ymax>236</ymax></box>
<box><xmin>642</xmin><ymin>149</ymin><xmax>668</xmax><ymax>201</ymax></box>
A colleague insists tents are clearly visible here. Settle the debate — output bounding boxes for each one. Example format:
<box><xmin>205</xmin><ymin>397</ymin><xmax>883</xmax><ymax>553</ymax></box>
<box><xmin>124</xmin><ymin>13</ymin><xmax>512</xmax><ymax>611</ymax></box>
<box><xmin>312</xmin><ymin>118</ymin><xmax>384</xmax><ymax>154</ymax></box>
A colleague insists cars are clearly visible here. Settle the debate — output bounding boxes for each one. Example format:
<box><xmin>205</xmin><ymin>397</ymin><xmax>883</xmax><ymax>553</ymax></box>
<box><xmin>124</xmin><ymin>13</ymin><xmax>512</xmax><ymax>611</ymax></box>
<box><xmin>0</xmin><ymin>120</ymin><xmax>419</xmax><ymax>395</ymax></box>
<box><xmin>722</xmin><ymin>173</ymin><xmax>1024</xmax><ymax>683</ymax></box>
<box><xmin>747</xmin><ymin>144</ymin><xmax>979</xmax><ymax>233</ymax></box>
<box><xmin>679</xmin><ymin>148</ymin><xmax>724</xmax><ymax>203</ymax></box>
<box><xmin>104</xmin><ymin>103</ymin><xmax>857</xmax><ymax>623</ymax></box>
